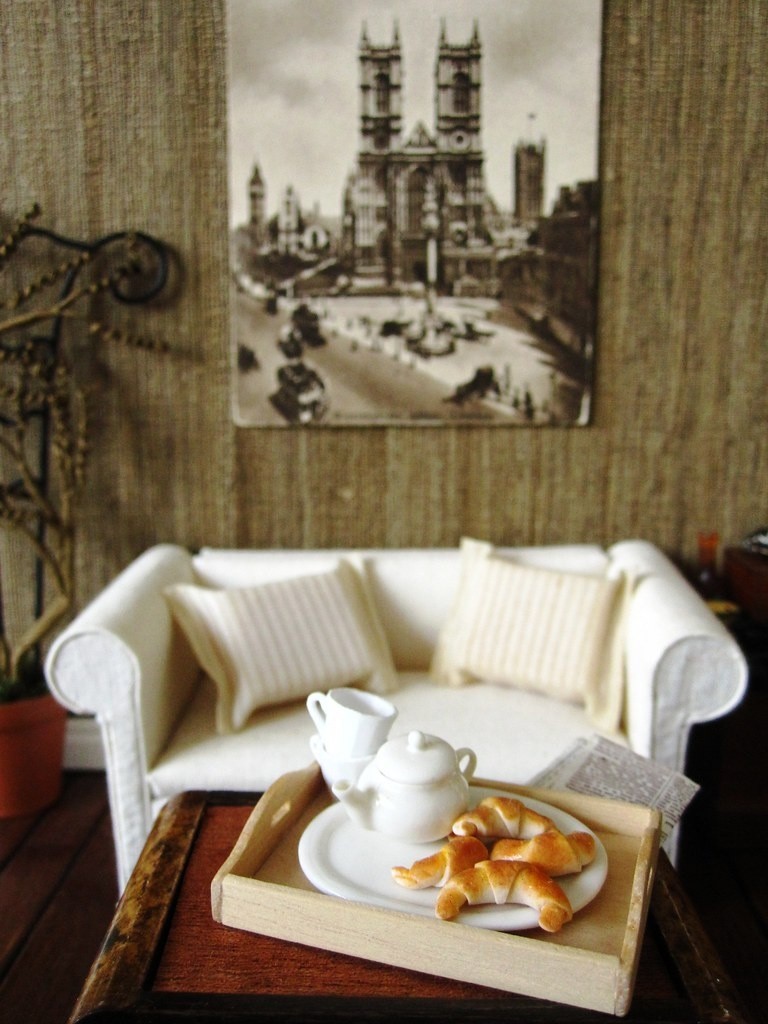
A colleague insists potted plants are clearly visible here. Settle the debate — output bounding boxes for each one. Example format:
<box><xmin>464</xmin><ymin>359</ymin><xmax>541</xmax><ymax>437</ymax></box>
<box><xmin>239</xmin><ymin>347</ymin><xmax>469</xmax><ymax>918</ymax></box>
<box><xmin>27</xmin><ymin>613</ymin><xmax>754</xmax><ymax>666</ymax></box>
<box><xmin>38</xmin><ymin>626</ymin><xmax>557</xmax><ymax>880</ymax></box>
<box><xmin>0</xmin><ymin>642</ymin><xmax>70</xmax><ymax>818</ymax></box>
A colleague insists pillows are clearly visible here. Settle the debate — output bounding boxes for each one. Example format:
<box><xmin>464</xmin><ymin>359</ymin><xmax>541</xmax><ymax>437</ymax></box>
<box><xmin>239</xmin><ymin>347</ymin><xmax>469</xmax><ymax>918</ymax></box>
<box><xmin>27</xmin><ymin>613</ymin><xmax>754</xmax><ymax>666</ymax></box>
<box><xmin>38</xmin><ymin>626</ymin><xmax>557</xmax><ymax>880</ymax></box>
<box><xmin>162</xmin><ymin>555</ymin><xmax>401</xmax><ymax>733</ymax></box>
<box><xmin>430</xmin><ymin>537</ymin><xmax>637</xmax><ymax>735</ymax></box>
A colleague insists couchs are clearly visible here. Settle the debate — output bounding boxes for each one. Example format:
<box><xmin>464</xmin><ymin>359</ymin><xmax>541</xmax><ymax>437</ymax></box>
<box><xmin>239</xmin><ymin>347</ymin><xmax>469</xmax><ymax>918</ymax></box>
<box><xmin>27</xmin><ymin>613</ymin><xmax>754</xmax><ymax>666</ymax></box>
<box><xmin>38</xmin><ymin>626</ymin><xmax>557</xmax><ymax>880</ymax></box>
<box><xmin>44</xmin><ymin>543</ymin><xmax>749</xmax><ymax>905</ymax></box>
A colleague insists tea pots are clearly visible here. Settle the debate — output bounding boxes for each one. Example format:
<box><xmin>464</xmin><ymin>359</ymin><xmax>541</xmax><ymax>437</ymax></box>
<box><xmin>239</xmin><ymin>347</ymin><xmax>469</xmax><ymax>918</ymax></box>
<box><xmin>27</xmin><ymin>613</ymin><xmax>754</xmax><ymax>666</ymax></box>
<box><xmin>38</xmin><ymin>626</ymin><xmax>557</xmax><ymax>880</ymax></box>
<box><xmin>330</xmin><ymin>731</ymin><xmax>477</xmax><ymax>843</ymax></box>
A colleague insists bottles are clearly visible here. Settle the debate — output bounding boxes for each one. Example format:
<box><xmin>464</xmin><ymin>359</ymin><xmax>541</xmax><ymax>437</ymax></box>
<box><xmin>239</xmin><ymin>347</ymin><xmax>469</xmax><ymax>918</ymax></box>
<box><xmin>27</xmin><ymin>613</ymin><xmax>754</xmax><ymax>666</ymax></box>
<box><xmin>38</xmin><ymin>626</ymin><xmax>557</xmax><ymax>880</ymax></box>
<box><xmin>693</xmin><ymin>530</ymin><xmax>723</xmax><ymax>599</ymax></box>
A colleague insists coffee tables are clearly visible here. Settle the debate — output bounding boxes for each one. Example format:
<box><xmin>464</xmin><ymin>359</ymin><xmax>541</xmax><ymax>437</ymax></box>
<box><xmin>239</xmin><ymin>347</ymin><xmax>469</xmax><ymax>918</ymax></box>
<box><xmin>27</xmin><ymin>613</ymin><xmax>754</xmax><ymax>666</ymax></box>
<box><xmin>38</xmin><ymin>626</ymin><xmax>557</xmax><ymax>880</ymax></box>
<box><xmin>71</xmin><ymin>791</ymin><xmax>758</xmax><ymax>1024</ymax></box>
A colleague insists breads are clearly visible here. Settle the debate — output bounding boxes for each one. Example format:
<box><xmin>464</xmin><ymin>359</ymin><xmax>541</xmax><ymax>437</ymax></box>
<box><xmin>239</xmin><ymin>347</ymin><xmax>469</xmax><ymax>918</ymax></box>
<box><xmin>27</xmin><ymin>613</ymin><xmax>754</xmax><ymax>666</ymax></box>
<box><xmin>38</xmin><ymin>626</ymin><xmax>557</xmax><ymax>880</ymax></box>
<box><xmin>392</xmin><ymin>795</ymin><xmax>596</xmax><ymax>932</ymax></box>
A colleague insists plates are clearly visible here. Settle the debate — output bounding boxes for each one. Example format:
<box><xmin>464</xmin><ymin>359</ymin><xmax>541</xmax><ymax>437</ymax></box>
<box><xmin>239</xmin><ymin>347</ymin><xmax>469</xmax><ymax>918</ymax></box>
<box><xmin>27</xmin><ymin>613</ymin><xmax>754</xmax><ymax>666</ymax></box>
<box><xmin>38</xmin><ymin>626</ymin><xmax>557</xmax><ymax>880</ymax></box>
<box><xmin>297</xmin><ymin>785</ymin><xmax>609</xmax><ymax>930</ymax></box>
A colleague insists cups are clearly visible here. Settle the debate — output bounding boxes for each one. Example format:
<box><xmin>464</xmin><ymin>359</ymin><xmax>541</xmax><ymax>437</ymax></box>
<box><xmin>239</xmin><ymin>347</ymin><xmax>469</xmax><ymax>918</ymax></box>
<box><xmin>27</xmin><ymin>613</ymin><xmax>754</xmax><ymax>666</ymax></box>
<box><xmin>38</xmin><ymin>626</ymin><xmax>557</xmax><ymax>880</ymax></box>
<box><xmin>305</xmin><ymin>686</ymin><xmax>399</xmax><ymax>757</ymax></box>
<box><xmin>308</xmin><ymin>734</ymin><xmax>374</xmax><ymax>796</ymax></box>
